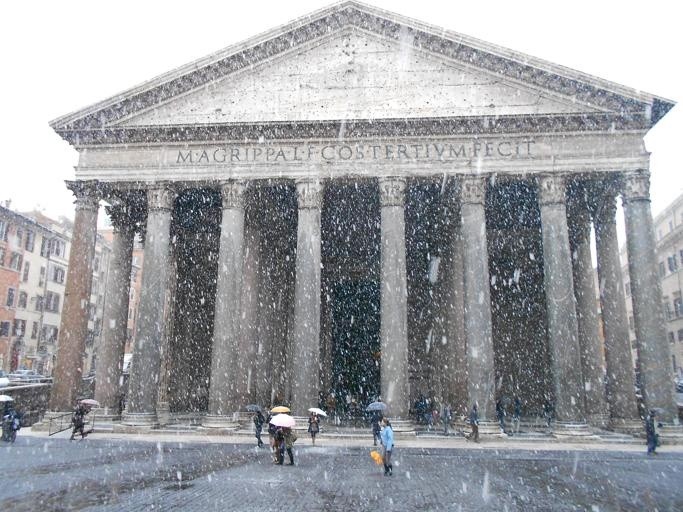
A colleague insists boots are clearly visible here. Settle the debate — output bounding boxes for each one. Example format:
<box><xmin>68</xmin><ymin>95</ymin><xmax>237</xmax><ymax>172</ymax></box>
<box><xmin>387</xmin><ymin>464</ymin><xmax>392</xmax><ymax>475</ymax></box>
<box><xmin>383</xmin><ymin>464</ymin><xmax>388</xmax><ymax>476</ymax></box>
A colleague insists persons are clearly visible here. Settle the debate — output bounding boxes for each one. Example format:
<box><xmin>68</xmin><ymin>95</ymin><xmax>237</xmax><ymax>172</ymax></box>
<box><xmin>265</xmin><ymin>411</ymin><xmax>297</xmax><ymax>466</ymax></box>
<box><xmin>368</xmin><ymin>409</ymin><xmax>383</xmax><ymax>446</ymax></box>
<box><xmin>251</xmin><ymin>410</ymin><xmax>265</xmax><ymax>449</ymax></box>
<box><xmin>414</xmin><ymin>392</ymin><xmax>559</xmax><ymax>444</ymax></box>
<box><xmin>647</xmin><ymin>409</ymin><xmax>661</xmax><ymax>447</ymax></box>
<box><xmin>306</xmin><ymin>412</ymin><xmax>320</xmax><ymax>446</ymax></box>
<box><xmin>3</xmin><ymin>407</ymin><xmax>16</xmax><ymax>442</ymax></box>
<box><xmin>379</xmin><ymin>418</ymin><xmax>394</xmax><ymax>476</ymax></box>
<box><xmin>645</xmin><ymin>414</ymin><xmax>658</xmax><ymax>455</ymax></box>
<box><xmin>70</xmin><ymin>407</ymin><xmax>86</xmax><ymax>439</ymax></box>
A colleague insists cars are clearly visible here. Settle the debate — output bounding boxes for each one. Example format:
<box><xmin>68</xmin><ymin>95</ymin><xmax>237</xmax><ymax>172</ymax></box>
<box><xmin>0</xmin><ymin>369</ymin><xmax>94</xmax><ymax>428</ymax></box>
<box><xmin>629</xmin><ymin>377</ymin><xmax>683</xmax><ymax>426</ymax></box>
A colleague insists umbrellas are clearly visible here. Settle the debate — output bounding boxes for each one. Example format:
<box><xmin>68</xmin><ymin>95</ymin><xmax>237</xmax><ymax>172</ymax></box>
<box><xmin>365</xmin><ymin>401</ymin><xmax>387</xmax><ymax>412</ymax></box>
<box><xmin>269</xmin><ymin>405</ymin><xmax>291</xmax><ymax>413</ymax></box>
<box><xmin>244</xmin><ymin>404</ymin><xmax>265</xmax><ymax>413</ymax></box>
<box><xmin>79</xmin><ymin>398</ymin><xmax>100</xmax><ymax>408</ymax></box>
<box><xmin>370</xmin><ymin>451</ymin><xmax>383</xmax><ymax>466</ymax></box>
<box><xmin>0</xmin><ymin>394</ymin><xmax>13</xmax><ymax>403</ymax></box>
<box><xmin>306</xmin><ymin>407</ymin><xmax>329</xmax><ymax>420</ymax></box>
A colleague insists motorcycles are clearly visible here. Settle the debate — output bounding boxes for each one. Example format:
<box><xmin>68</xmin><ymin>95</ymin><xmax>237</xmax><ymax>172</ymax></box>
<box><xmin>1</xmin><ymin>417</ymin><xmax>20</xmax><ymax>443</ymax></box>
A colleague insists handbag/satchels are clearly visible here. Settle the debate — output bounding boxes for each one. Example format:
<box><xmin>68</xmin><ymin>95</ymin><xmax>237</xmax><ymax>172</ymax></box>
<box><xmin>290</xmin><ymin>431</ymin><xmax>297</xmax><ymax>442</ymax></box>
